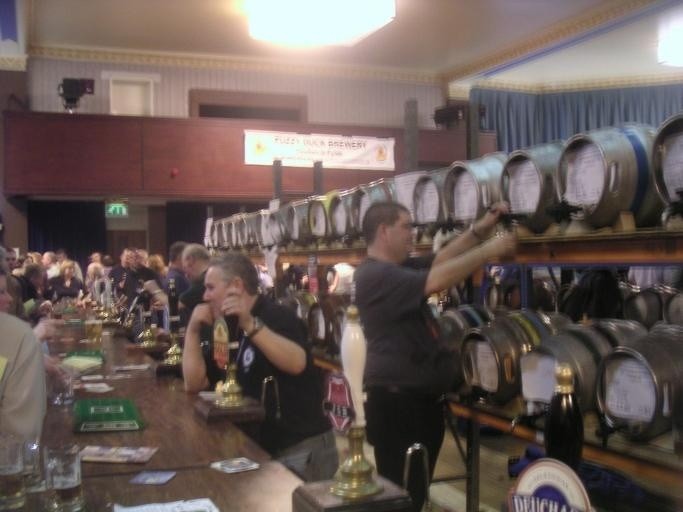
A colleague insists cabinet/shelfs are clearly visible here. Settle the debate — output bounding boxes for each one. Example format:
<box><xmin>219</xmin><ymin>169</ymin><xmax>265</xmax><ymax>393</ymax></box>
<box><xmin>230</xmin><ymin>215</ymin><xmax>682</xmax><ymax>512</ymax></box>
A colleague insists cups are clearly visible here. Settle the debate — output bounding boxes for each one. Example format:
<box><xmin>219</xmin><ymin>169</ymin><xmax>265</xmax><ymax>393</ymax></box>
<box><xmin>46</xmin><ymin>369</ymin><xmax>74</xmax><ymax>406</ymax></box>
<box><xmin>0</xmin><ymin>433</ymin><xmax>86</xmax><ymax>512</ymax></box>
<box><xmin>85</xmin><ymin>318</ymin><xmax>104</xmax><ymax>354</ymax></box>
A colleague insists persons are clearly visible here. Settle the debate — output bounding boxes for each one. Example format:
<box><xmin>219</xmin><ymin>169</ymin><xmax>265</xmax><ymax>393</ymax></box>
<box><xmin>575</xmin><ymin>267</ymin><xmax>624</xmax><ymax>323</ymax></box>
<box><xmin>181</xmin><ymin>252</ymin><xmax>339</xmax><ymax>482</ymax></box>
<box><xmin>0</xmin><ymin>244</ymin><xmax>84</xmax><ymax>479</ymax></box>
<box><xmin>84</xmin><ymin>241</ymin><xmax>213</xmax><ymax>331</ymax></box>
<box><xmin>352</xmin><ymin>200</ymin><xmax>517</xmax><ymax>511</ymax></box>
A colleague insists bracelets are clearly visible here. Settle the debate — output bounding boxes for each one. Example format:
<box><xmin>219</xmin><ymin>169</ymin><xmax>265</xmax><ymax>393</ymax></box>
<box><xmin>468</xmin><ymin>223</ymin><xmax>487</xmax><ymax>240</ymax></box>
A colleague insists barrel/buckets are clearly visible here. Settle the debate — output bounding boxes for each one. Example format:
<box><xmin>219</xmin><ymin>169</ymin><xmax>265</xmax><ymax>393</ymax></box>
<box><xmin>650</xmin><ymin>114</ymin><xmax>683</xmax><ymax>213</ymax></box>
<box><xmin>444</xmin><ymin>150</ymin><xmax>507</xmax><ymax>228</ymax></box>
<box><xmin>511</xmin><ymin>456</ymin><xmax>644</xmax><ymax>512</ymax></box>
<box><xmin>556</xmin><ymin>120</ymin><xmax>662</xmax><ymax>230</ymax></box>
<box><xmin>435</xmin><ymin>263</ymin><xmax>681</xmax><ymax>456</ymax></box>
<box><xmin>210</xmin><ymin>162</ymin><xmax>443</xmax><ymax>253</ymax></box>
<box><xmin>499</xmin><ymin>135</ymin><xmax>570</xmax><ymax>235</ymax></box>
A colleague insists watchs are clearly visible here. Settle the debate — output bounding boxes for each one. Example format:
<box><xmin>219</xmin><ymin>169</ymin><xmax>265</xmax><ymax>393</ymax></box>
<box><xmin>244</xmin><ymin>317</ymin><xmax>263</xmax><ymax>337</ymax></box>
